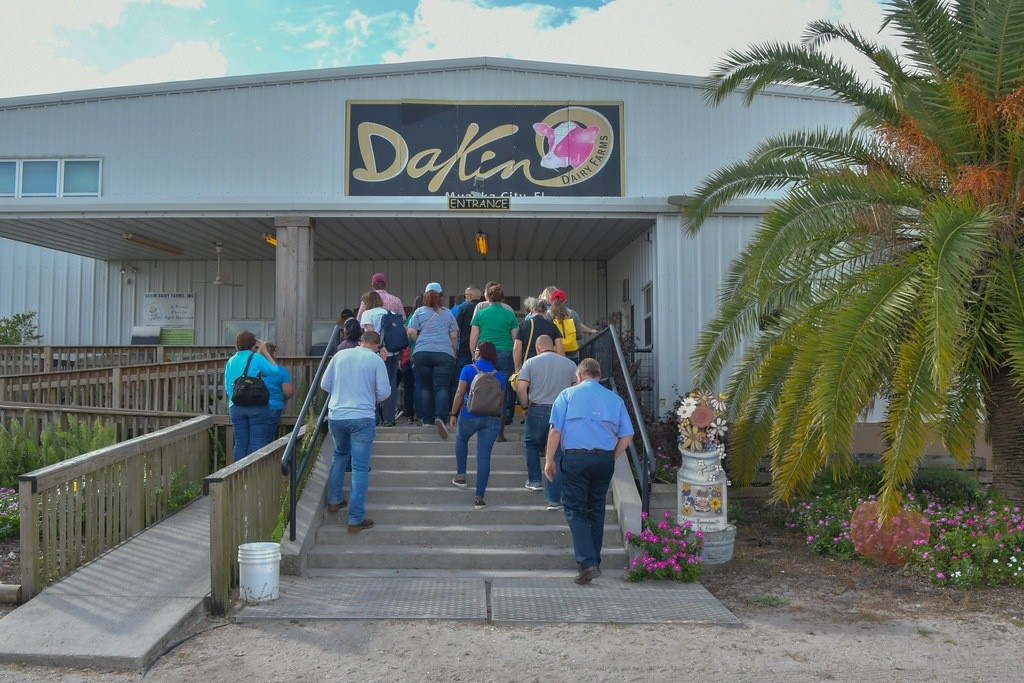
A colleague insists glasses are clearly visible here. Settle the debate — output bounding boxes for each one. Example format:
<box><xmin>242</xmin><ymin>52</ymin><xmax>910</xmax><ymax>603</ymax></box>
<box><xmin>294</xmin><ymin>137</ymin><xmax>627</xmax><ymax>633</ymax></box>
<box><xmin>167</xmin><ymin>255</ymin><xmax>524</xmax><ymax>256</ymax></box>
<box><xmin>464</xmin><ymin>293</ymin><xmax>473</xmax><ymax>298</ymax></box>
<box><xmin>474</xmin><ymin>350</ymin><xmax>480</xmax><ymax>352</ymax></box>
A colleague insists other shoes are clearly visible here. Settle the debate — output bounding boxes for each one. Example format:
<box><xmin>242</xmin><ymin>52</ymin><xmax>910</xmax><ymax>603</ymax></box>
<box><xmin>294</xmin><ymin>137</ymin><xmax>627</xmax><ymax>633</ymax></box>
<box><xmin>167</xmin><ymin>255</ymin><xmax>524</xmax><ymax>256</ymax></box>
<box><xmin>403</xmin><ymin>418</ymin><xmax>414</xmax><ymax>424</ymax></box>
<box><xmin>383</xmin><ymin>420</ymin><xmax>393</xmax><ymax>426</ymax></box>
<box><xmin>435</xmin><ymin>416</ymin><xmax>448</xmax><ymax>439</ymax></box>
<box><xmin>422</xmin><ymin>417</ymin><xmax>434</xmax><ymax>427</ymax></box>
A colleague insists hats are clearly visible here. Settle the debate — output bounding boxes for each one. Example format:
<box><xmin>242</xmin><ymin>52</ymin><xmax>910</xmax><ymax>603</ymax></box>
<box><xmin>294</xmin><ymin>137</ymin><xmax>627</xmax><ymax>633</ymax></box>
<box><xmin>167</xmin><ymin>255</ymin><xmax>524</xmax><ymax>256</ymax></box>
<box><xmin>551</xmin><ymin>289</ymin><xmax>567</xmax><ymax>302</ymax></box>
<box><xmin>425</xmin><ymin>282</ymin><xmax>443</xmax><ymax>294</ymax></box>
<box><xmin>371</xmin><ymin>273</ymin><xmax>388</xmax><ymax>285</ymax></box>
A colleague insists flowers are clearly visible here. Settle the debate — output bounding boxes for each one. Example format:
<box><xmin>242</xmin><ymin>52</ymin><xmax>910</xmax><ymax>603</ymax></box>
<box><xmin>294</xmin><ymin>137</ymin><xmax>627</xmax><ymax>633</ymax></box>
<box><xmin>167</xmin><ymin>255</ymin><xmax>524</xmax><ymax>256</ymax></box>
<box><xmin>675</xmin><ymin>390</ymin><xmax>729</xmax><ymax>454</ymax></box>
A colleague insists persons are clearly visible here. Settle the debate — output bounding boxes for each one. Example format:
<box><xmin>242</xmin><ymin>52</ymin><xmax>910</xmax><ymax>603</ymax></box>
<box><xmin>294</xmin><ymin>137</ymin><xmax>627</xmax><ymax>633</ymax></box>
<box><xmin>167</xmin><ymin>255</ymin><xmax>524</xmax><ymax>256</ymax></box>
<box><xmin>518</xmin><ymin>335</ymin><xmax>578</xmax><ymax>509</ymax></box>
<box><xmin>544</xmin><ymin>359</ymin><xmax>634</xmax><ymax>585</ymax></box>
<box><xmin>320</xmin><ymin>329</ymin><xmax>374</xmax><ymax>532</ymax></box>
<box><xmin>449</xmin><ymin>339</ymin><xmax>506</xmax><ymax>504</ymax></box>
<box><xmin>333</xmin><ymin>273</ymin><xmax>601</xmax><ymax>440</ymax></box>
<box><xmin>224</xmin><ymin>330</ymin><xmax>279</xmax><ymax>465</ymax></box>
<box><xmin>257</xmin><ymin>339</ymin><xmax>296</xmax><ymax>443</ymax></box>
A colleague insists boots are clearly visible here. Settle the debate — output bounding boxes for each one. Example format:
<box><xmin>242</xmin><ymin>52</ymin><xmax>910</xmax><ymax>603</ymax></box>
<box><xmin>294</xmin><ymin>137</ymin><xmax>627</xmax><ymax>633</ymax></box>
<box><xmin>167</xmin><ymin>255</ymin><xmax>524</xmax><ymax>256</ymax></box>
<box><xmin>496</xmin><ymin>414</ymin><xmax>508</xmax><ymax>442</ymax></box>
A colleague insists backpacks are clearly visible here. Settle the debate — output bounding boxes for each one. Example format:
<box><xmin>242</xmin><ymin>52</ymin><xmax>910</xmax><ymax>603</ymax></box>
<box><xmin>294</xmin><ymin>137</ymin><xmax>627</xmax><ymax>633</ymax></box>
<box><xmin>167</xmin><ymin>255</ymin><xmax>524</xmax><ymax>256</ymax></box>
<box><xmin>467</xmin><ymin>364</ymin><xmax>505</xmax><ymax>416</ymax></box>
<box><xmin>553</xmin><ymin>318</ymin><xmax>579</xmax><ymax>352</ymax></box>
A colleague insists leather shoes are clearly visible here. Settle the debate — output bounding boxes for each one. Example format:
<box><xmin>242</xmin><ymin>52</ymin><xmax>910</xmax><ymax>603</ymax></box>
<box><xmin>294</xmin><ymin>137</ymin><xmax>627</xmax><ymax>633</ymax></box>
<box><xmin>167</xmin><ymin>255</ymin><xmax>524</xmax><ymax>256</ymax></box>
<box><xmin>574</xmin><ymin>565</ymin><xmax>601</xmax><ymax>583</ymax></box>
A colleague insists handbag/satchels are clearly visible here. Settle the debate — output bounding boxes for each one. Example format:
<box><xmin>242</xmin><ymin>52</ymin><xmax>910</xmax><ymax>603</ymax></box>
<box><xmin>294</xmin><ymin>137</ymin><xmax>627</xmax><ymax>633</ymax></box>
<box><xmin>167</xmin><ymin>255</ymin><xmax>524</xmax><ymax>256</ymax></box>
<box><xmin>232</xmin><ymin>377</ymin><xmax>270</xmax><ymax>406</ymax></box>
<box><xmin>509</xmin><ymin>372</ymin><xmax>521</xmax><ymax>391</ymax></box>
<box><xmin>382</xmin><ymin>310</ymin><xmax>408</xmax><ymax>351</ymax></box>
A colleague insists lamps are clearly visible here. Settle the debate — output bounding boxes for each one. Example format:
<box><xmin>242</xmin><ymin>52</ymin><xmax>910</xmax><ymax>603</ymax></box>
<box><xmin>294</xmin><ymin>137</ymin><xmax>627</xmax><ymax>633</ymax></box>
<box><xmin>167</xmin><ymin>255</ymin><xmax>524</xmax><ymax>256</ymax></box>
<box><xmin>265</xmin><ymin>233</ymin><xmax>278</xmax><ymax>246</ymax></box>
<box><xmin>123</xmin><ymin>234</ymin><xmax>176</xmax><ymax>254</ymax></box>
<box><xmin>475</xmin><ymin>232</ymin><xmax>489</xmax><ymax>256</ymax></box>
<box><xmin>120</xmin><ymin>264</ymin><xmax>137</xmax><ymax>274</ymax></box>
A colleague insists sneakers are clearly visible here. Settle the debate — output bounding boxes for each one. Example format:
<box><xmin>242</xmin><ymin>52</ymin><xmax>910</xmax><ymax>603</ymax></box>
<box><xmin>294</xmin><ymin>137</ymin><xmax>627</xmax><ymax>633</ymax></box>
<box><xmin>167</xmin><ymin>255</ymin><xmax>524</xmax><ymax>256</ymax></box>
<box><xmin>525</xmin><ymin>480</ymin><xmax>543</xmax><ymax>490</ymax></box>
<box><xmin>348</xmin><ymin>519</ymin><xmax>374</xmax><ymax>532</ymax></box>
<box><xmin>328</xmin><ymin>499</ymin><xmax>347</xmax><ymax>512</ymax></box>
<box><xmin>547</xmin><ymin>501</ymin><xmax>563</xmax><ymax>510</ymax></box>
<box><xmin>474</xmin><ymin>500</ymin><xmax>487</xmax><ymax>509</ymax></box>
<box><xmin>453</xmin><ymin>477</ymin><xmax>467</xmax><ymax>487</ymax></box>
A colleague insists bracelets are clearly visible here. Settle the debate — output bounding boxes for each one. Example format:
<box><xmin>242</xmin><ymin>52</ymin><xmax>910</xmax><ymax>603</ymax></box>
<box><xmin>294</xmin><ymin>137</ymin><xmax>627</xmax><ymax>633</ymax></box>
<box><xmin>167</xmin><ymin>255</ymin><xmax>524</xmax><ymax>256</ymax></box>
<box><xmin>449</xmin><ymin>413</ymin><xmax>457</xmax><ymax>416</ymax></box>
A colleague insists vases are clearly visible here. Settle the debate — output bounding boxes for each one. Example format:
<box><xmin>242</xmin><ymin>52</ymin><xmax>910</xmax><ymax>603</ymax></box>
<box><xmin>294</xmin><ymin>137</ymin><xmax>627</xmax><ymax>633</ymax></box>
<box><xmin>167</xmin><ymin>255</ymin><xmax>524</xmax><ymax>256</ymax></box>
<box><xmin>676</xmin><ymin>442</ymin><xmax>728</xmax><ymax>531</ymax></box>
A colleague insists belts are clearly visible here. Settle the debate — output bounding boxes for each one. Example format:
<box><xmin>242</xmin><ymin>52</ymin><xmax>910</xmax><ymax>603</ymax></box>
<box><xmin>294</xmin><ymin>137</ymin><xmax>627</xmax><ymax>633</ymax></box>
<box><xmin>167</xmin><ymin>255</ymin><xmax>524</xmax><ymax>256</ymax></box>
<box><xmin>564</xmin><ymin>448</ymin><xmax>614</xmax><ymax>454</ymax></box>
<box><xmin>387</xmin><ymin>351</ymin><xmax>399</xmax><ymax>357</ymax></box>
<box><xmin>530</xmin><ymin>403</ymin><xmax>552</xmax><ymax>407</ymax></box>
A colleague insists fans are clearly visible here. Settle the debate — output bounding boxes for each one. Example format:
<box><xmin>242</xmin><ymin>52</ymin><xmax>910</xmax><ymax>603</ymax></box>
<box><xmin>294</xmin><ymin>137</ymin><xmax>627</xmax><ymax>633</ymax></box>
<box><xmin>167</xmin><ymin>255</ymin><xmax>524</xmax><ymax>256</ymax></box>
<box><xmin>190</xmin><ymin>241</ymin><xmax>243</xmax><ymax>287</ymax></box>
